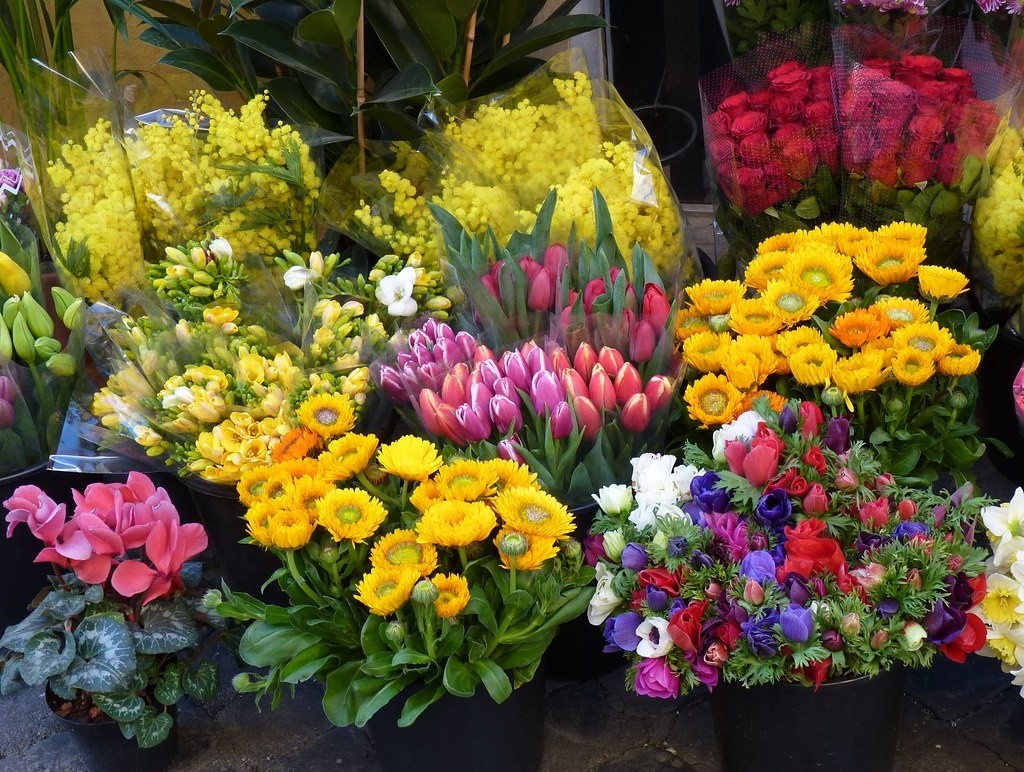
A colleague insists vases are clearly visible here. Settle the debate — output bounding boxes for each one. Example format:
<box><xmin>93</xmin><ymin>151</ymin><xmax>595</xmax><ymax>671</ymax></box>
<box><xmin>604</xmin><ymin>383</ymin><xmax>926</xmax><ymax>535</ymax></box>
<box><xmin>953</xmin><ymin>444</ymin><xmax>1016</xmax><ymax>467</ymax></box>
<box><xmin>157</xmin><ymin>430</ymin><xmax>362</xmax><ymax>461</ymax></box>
<box><xmin>0</xmin><ymin>208</ymin><xmax>1024</xmax><ymax>772</ymax></box>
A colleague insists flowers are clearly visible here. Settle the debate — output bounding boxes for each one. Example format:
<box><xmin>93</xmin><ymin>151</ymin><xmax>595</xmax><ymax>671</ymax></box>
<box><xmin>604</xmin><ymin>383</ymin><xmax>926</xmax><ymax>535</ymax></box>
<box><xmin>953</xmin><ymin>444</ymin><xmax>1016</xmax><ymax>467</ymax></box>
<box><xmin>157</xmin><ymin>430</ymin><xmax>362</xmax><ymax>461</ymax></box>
<box><xmin>0</xmin><ymin>0</ymin><xmax>1024</xmax><ymax>751</ymax></box>
